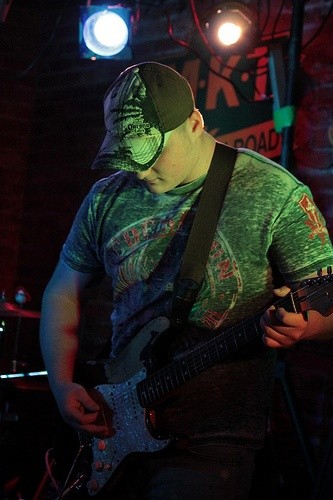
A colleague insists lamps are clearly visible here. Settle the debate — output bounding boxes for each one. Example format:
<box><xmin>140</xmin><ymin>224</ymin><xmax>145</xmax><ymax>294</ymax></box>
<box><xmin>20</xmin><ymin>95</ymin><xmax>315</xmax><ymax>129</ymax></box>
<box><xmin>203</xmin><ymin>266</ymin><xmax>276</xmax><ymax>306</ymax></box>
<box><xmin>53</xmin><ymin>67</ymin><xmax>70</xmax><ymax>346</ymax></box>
<box><xmin>78</xmin><ymin>0</ymin><xmax>140</xmax><ymax>60</ymax></box>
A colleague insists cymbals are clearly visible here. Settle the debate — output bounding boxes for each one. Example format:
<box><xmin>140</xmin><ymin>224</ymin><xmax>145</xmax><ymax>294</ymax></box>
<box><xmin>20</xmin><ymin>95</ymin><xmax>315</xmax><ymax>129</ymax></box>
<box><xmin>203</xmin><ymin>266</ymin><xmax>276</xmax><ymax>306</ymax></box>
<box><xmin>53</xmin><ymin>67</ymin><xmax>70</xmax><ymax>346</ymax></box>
<box><xmin>0</xmin><ymin>299</ymin><xmax>41</xmax><ymax>319</ymax></box>
<box><xmin>0</xmin><ymin>377</ymin><xmax>51</xmax><ymax>391</ymax></box>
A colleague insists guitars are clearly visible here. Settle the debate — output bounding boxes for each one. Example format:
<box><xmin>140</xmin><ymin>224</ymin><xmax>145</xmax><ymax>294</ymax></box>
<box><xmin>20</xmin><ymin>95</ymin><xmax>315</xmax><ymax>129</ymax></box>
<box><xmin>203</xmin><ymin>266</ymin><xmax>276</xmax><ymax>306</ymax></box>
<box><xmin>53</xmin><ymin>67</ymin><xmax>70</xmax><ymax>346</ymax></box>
<box><xmin>86</xmin><ymin>266</ymin><xmax>333</xmax><ymax>498</ymax></box>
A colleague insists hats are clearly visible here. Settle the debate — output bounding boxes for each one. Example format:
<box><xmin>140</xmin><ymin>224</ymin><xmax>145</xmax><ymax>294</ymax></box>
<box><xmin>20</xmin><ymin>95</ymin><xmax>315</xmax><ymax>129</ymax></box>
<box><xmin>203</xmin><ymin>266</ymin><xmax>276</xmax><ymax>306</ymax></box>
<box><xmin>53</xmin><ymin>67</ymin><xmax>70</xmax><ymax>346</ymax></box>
<box><xmin>90</xmin><ymin>62</ymin><xmax>195</xmax><ymax>173</ymax></box>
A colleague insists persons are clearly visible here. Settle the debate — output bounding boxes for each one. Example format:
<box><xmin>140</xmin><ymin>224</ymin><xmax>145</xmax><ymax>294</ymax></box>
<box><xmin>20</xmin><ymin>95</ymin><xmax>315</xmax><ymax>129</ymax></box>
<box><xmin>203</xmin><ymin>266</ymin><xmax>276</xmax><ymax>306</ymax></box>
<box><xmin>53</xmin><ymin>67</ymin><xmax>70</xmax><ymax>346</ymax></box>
<box><xmin>38</xmin><ymin>61</ymin><xmax>333</xmax><ymax>500</ymax></box>
